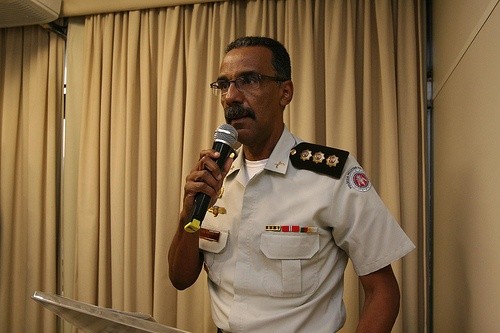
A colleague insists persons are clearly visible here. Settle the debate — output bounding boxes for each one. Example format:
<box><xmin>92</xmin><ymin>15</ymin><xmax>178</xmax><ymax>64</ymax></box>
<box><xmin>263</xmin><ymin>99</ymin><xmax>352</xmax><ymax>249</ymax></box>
<box><xmin>167</xmin><ymin>35</ymin><xmax>419</xmax><ymax>332</ymax></box>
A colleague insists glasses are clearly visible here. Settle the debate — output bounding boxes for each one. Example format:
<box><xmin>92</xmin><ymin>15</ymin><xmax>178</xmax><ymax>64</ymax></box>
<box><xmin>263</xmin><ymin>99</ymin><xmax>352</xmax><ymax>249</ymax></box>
<box><xmin>210</xmin><ymin>73</ymin><xmax>289</xmax><ymax>96</ymax></box>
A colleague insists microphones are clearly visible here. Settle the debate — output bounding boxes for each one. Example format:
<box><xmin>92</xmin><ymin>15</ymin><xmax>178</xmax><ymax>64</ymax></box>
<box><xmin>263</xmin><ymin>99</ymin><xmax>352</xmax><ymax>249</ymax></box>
<box><xmin>184</xmin><ymin>124</ymin><xmax>239</xmax><ymax>233</ymax></box>
